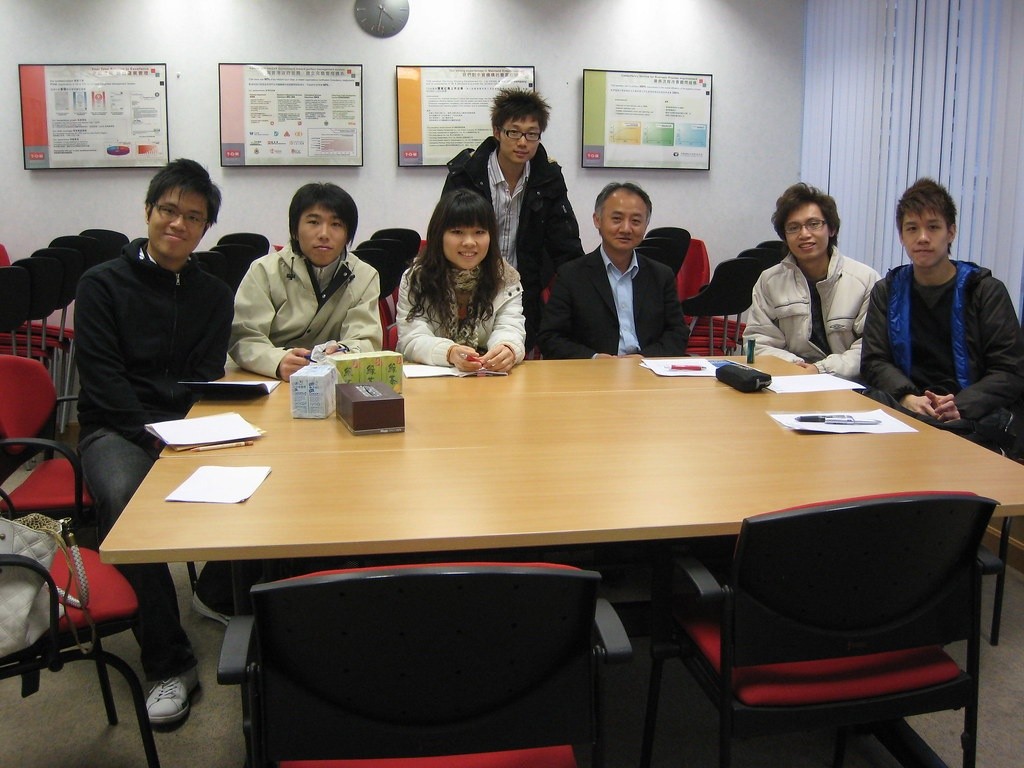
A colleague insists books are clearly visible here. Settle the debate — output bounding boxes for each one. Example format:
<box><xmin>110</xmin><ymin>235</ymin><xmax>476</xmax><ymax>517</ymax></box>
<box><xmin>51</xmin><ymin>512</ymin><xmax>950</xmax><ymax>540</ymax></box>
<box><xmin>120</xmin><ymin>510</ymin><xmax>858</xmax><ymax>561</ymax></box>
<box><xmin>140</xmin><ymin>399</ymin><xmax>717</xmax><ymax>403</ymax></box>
<box><xmin>144</xmin><ymin>381</ymin><xmax>282</xmax><ymax>394</ymax></box>
<box><xmin>145</xmin><ymin>411</ymin><xmax>267</xmax><ymax>452</ymax></box>
<box><xmin>402</xmin><ymin>365</ymin><xmax>508</xmax><ymax>378</ymax></box>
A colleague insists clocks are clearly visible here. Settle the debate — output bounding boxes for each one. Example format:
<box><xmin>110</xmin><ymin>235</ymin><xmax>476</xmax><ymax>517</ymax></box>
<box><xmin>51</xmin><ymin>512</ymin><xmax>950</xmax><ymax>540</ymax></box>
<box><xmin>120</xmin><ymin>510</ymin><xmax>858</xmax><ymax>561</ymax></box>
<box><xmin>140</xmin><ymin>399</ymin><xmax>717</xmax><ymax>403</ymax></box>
<box><xmin>353</xmin><ymin>0</ymin><xmax>409</xmax><ymax>39</ymax></box>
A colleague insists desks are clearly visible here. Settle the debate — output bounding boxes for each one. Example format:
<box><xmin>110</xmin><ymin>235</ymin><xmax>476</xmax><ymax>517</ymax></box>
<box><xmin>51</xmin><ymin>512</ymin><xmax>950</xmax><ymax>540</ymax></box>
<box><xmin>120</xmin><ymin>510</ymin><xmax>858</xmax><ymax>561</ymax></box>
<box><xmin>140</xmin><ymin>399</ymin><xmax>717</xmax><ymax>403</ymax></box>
<box><xmin>98</xmin><ymin>354</ymin><xmax>1024</xmax><ymax>615</ymax></box>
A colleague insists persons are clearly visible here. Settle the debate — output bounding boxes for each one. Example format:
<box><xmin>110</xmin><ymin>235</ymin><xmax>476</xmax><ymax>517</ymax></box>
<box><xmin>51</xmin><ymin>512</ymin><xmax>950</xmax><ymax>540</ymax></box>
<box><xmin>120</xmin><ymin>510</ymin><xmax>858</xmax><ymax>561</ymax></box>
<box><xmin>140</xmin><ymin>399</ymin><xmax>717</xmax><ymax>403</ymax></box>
<box><xmin>860</xmin><ymin>177</ymin><xmax>1024</xmax><ymax>461</ymax></box>
<box><xmin>536</xmin><ymin>181</ymin><xmax>689</xmax><ymax>359</ymax></box>
<box><xmin>743</xmin><ymin>182</ymin><xmax>882</xmax><ymax>395</ymax></box>
<box><xmin>73</xmin><ymin>157</ymin><xmax>235</xmax><ymax>724</ymax></box>
<box><xmin>396</xmin><ymin>188</ymin><xmax>527</xmax><ymax>373</ymax></box>
<box><xmin>228</xmin><ymin>183</ymin><xmax>383</xmax><ymax>383</ymax></box>
<box><xmin>440</xmin><ymin>86</ymin><xmax>586</xmax><ymax>288</ymax></box>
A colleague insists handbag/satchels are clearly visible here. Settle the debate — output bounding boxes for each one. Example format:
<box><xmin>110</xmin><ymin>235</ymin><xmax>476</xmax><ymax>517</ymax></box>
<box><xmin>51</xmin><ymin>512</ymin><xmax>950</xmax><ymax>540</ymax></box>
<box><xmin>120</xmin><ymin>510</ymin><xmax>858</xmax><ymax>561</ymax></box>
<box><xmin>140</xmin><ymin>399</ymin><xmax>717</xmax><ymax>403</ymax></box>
<box><xmin>0</xmin><ymin>512</ymin><xmax>90</xmax><ymax>659</ymax></box>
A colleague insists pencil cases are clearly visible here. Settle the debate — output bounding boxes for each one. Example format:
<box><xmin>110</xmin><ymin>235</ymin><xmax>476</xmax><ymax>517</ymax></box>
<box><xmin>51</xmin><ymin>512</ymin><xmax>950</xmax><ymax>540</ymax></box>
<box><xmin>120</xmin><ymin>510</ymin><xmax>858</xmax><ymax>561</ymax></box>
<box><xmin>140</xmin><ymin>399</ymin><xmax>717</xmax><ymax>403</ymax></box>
<box><xmin>715</xmin><ymin>364</ymin><xmax>773</xmax><ymax>394</ymax></box>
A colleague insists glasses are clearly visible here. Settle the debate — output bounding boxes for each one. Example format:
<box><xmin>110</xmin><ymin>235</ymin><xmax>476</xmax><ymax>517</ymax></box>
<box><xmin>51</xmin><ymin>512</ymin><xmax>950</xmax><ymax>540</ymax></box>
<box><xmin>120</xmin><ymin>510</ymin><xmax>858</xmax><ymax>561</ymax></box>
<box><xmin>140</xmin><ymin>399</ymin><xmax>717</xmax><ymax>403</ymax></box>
<box><xmin>785</xmin><ymin>219</ymin><xmax>830</xmax><ymax>234</ymax></box>
<box><xmin>147</xmin><ymin>199</ymin><xmax>210</xmax><ymax>225</ymax></box>
<box><xmin>497</xmin><ymin>124</ymin><xmax>542</xmax><ymax>141</ymax></box>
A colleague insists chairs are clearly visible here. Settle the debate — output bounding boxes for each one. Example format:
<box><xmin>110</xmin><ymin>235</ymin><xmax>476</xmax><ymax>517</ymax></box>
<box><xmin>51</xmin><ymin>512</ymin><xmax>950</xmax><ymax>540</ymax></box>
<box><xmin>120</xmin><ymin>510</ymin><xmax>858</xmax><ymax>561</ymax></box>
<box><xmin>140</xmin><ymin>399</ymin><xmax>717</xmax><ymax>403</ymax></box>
<box><xmin>0</xmin><ymin>227</ymin><xmax>1024</xmax><ymax>768</ymax></box>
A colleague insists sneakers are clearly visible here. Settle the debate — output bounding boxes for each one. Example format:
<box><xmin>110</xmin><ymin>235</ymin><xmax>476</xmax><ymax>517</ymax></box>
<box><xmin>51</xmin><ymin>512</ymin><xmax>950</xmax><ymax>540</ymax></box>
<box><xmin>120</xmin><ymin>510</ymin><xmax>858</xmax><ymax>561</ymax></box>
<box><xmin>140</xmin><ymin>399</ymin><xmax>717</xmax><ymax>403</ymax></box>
<box><xmin>145</xmin><ymin>666</ymin><xmax>202</xmax><ymax>723</ymax></box>
<box><xmin>193</xmin><ymin>591</ymin><xmax>231</xmax><ymax>627</ymax></box>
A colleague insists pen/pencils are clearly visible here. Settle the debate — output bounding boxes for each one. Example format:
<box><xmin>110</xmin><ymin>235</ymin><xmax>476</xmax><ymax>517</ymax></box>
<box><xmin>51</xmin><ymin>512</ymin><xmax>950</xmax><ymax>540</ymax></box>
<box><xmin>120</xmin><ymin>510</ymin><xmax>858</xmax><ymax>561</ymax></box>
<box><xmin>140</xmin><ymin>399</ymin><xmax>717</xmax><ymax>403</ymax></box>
<box><xmin>460</xmin><ymin>353</ymin><xmax>484</xmax><ymax>366</ymax></box>
<box><xmin>794</xmin><ymin>415</ymin><xmax>882</xmax><ymax>426</ymax></box>
<box><xmin>305</xmin><ymin>348</ymin><xmax>347</xmax><ymax>360</ymax></box>
<box><xmin>190</xmin><ymin>441</ymin><xmax>255</xmax><ymax>453</ymax></box>
<box><xmin>671</xmin><ymin>365</ymin><xmax>706</xmax><ymax>371</ymax></box>
